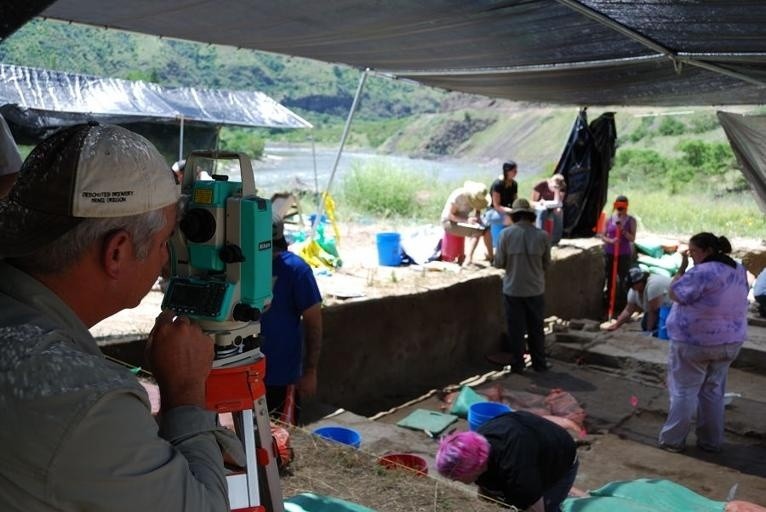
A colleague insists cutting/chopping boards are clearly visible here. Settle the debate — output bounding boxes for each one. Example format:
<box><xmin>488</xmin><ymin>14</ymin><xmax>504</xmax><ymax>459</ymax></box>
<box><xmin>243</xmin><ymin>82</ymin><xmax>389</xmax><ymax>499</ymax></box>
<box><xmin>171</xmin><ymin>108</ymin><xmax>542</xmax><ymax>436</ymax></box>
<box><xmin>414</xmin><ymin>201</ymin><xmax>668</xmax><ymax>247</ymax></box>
<box><xmin>398</xmin><ymin>408</ymin><xmax>458</xmax><ymax>433</ymax></box>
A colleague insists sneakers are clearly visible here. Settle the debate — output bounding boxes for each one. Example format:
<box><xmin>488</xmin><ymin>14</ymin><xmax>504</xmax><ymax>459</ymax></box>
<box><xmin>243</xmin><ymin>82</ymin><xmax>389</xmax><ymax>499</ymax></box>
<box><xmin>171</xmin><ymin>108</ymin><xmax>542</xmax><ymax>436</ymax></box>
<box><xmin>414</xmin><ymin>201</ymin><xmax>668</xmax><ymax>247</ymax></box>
<box><xmin>665</xmin><ymin>444</ymin><xmax>722</xmax><ymax>454</ymax></box>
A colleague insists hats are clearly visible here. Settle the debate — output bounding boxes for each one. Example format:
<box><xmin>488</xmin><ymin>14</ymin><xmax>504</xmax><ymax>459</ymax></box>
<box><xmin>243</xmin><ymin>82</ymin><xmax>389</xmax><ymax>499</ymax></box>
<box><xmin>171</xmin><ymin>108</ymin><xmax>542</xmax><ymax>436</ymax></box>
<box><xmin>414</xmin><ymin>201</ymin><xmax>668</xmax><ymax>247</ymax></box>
<box><xmin>463</xmin><ymin>180</ymin><xmax>493</xmax><ymax>209</ymax></box>
<box><xmin>272</xmin><ymin>213</ymin><xmax>284</xmax><ymax>241</ymax></box>
<box><xmin>6</xmin><ymin>119</ymin><xmax>182</xmax><ymax>220</ymax></box>
<box><xmin>546</xmin><ymin>173</ymin><xmax>566</xmax><ymax>189</ymax></box>
<box><xmin>504</xmin><ymin>197</ymin><xmax>537</xmax><ymax>214</ymax></box>
<box><xmin>624</xmin><ymin>266</ymin><xmax>644</xmax><ymax>283</ymax></box>
<box><xmin>614</xmin><ymin>195</ymin><xmax>629</xmax><ymax>209</ymax></box>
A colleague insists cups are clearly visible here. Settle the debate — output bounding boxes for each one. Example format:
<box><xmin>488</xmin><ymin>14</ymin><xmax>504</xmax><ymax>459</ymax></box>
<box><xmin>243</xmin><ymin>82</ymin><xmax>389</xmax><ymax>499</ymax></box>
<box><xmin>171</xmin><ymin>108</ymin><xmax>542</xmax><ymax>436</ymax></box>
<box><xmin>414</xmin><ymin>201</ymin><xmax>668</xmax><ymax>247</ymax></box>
<box><xmin>313</xmin><ymin>426</ymin><xmax>362</xmax><ymax>451</ymax></box>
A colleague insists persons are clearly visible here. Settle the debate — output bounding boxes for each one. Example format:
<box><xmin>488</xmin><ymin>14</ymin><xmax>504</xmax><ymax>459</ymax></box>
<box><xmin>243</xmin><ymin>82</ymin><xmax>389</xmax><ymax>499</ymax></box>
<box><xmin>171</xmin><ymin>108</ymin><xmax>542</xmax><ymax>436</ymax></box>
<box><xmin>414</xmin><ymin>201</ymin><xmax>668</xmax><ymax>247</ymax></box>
<box><xmin>754</xmin><ymin>268</ymin><xmax>766</xmax><ymax>319</ymax></box>
<box><xmin>658</xmin><ymin>233</ymin><xmax>748</xmax><ymax>454</ymax></box>
<box><xmin>607</xmin><ymin>267</ymin><xmax>672</xmax><ymax>333</ymax></box>
<box><xmin>254</xmin><ymin>213</ymin><xmax>323</xmax><ymax>425</ymax></box>
<box><xmin>1</xmin><ymin>118</ymin><xmax>234</xmax><ymax>511</ymax></box>
<box><xmin>435</xmin><ymin>413</ymin><xmax>579</xmax><ymax>512</ymax></box>
<box><xmin>0</xmin><ymin>111</ymin><xmax>24</xmax><ymax>200</ymax></box>
<box><xmin>601</xmin><ymin>196</ymin><xmax>636</xmax><ymax>322</ymax></box>
<box><xmin>439</xmin><ymin>159</ymin><xmax>567</xmax><ymax>375</ymax></box>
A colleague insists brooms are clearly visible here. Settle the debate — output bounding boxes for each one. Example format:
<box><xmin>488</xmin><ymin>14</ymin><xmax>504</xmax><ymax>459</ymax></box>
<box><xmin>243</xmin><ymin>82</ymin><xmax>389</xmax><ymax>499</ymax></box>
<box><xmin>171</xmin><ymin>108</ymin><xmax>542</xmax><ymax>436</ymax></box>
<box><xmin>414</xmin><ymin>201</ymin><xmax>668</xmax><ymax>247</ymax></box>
<box><xmin>599</xmin><ymin>220</ymin><xmax>622</xmax><ymax>331</ymax></box>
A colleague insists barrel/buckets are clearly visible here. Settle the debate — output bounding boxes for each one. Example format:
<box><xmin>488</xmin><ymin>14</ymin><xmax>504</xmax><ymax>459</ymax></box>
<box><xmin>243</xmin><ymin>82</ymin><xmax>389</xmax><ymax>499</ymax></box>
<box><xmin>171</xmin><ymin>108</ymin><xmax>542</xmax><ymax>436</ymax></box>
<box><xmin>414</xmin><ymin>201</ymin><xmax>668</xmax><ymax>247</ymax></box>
<box><xmin>490</xmin><ymin>223</ymin><xmax>506</xmax><ymax>247</ymax></box>
<box><xmin>545</xmin><ymin>214</ymin><xmax>554</xmax><ymax>237</ymax></box>
<box><xmin>657</xmin><ymin>303</ymin><xmax>672</xmax><ymax>339</ymax></box>
<box><xmin>311</xmin><ymin>425</ymin><xmax>362</xmax><ymax>449</ymax></box>
<box><xmin>594</xmin><ymin>210</ymin><xmax>606</xmax><ymax>237</ymax></box>
<box><xmin>440</xmin><ymin>232</ymin><xmax>465</xmax><ymax>263</ymax></box>
